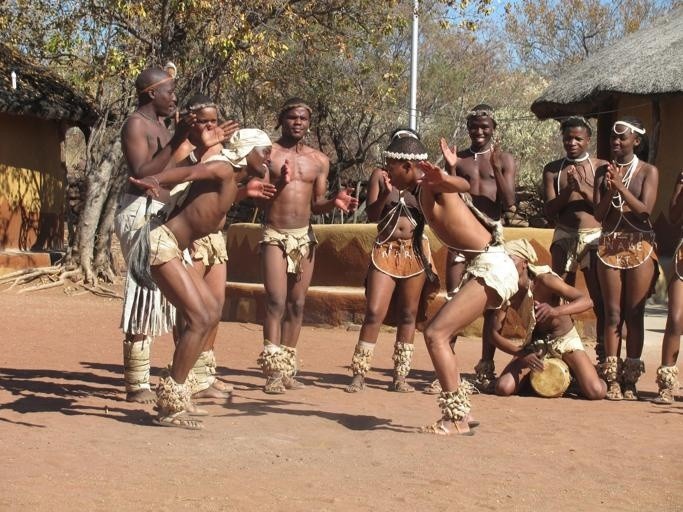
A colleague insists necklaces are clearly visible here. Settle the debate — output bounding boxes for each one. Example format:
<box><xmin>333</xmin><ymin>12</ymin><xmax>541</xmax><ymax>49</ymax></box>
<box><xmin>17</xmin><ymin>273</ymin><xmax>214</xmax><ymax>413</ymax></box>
<box><xmin>613</xmin><ymin>153</ymin><xmax>640</xmax><ymax>209</ymax></box>
<box><xmin>135</xmin><ymin>107</ymin><xmax>164</xmax><ymax>124</ymax></box>
<box><xmin>467</xmin><ymin>144</ymin><xmax>497</xmax><ymax>156</ymax></box>
<box><xmin>566</xmin><ymin>152</ymin><xmax>591</xmax><ymax>162</ymax></box>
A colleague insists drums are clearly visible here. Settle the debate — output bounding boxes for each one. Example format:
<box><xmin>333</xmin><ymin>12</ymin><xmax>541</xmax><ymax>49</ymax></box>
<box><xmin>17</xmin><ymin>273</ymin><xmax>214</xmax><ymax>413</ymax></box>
<box><xmin>529</xmin><ymin>358</ymin><xmax>572</xmax><ymax>398</ymax></box>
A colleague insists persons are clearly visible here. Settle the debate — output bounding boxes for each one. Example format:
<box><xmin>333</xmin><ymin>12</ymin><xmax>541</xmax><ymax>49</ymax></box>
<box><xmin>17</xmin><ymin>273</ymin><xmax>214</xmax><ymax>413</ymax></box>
<box><xmin>113</xmin><ymin>64</ymin><xmax>240</xmax><ymax>407</ymax></box>
<box><xmin>654</xmin><ymin>161</ymin><xmax>683</xmax><ymax>405</ymax></box>
<box><xmin>127</xmin><ymin>126</ymin><xmax>274</xmax><ymax>429</ymax></box>
<box><xmin>542</xmin><ymin>113</ymin><xmax>611</xmax><ymax>385</ymax></box>
<box><xmin>592</xmin><ymin>118</ymin><xmax>661</xmax><ymax>403</ymax></box>
<box><xmin>494</xmin><ymin>237</ymin><xmax>610</xmax><ymax>399</ymax></box>
<box><xmin>385</xmin><ymin>135</ymin><xmax>523</xmax><ymax>437</ymax></box>
<box><xmin>252</xmin><ymin>97</ymin><xmax>360</xmax><ymax>395</ymax></box>
<box><xmin>172</xmin><ymin>93</ymin><xmax>233</xmax><ymax>406</ymax></box>
<box><xmin>343</xmin><ymin>127</ymin><xmax>441</xmax><ymax>396</ymax></box>
<box><xmin>423</xmin><ymin>104</ymin><xmax>518</xmax><ymax>395</ymax></box>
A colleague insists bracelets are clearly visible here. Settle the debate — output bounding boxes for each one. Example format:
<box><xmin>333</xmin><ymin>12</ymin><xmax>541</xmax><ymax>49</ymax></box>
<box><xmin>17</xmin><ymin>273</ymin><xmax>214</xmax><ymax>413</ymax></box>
<box><xmin>188</xmin><ymin>150</ymin><xmax>200</xmax><ymax>163</ymax></box>
<box><xmin>147</xmin><ymin>174</ymin><xmax>161</xmax><ymax>186</ymax></box>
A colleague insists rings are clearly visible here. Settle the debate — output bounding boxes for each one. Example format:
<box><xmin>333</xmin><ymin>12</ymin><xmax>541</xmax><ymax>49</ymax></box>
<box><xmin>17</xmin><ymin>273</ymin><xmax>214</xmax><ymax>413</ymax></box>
<box><xmin>532</xmin><ymin>364</ymin><xmax>535</xmax><ymax>367</ymax></box>
